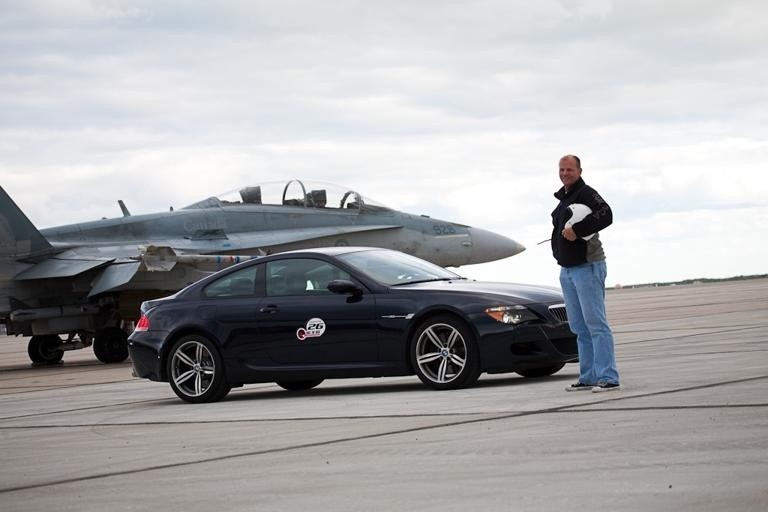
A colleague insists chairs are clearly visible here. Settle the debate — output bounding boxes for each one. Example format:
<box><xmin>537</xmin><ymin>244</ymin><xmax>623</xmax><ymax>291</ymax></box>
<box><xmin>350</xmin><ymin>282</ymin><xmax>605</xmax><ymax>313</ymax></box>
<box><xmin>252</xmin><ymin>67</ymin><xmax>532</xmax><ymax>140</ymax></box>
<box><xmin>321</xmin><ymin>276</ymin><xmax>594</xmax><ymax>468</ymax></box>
<box><xmin>230</xmin><ymin>277</ymin><xmax>254</xmax><ymax>295</ymax></box>
<box><xmin>283</xmin><ymin>267</ymin><xmax>307</xmax><ymax>291</ymax></box>
<box><xmin>271</xmin><ymin>275</ymin><xmax>286</xmax><ymax>295</ymax></box>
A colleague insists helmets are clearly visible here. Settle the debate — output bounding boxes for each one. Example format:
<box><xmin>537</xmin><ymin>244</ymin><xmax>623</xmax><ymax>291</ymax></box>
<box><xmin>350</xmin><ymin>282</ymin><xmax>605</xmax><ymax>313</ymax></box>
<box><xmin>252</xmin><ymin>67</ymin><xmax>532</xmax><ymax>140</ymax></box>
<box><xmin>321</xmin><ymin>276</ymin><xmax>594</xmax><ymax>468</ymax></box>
<box><xmin>564</xmin><ymin>203</ymin><xmax>596</xmax><ymax>241</ymax></box>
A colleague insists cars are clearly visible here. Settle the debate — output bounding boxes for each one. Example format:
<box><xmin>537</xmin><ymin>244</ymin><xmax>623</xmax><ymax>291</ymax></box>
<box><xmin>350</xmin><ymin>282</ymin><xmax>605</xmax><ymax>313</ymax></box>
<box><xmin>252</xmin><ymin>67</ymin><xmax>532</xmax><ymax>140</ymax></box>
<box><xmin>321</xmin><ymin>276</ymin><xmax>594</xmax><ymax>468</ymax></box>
<box><xmin>126</xmin><ymin>245</ymin><xmax>580</xmax><ymax>402</ymax></box>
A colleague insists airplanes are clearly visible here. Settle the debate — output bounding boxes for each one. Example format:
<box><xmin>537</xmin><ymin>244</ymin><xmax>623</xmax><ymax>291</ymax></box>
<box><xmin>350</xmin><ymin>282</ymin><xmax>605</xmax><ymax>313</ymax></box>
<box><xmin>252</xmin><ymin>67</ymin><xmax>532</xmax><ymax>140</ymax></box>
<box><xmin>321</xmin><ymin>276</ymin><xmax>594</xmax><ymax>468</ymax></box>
<box><xmin>0</xmin><ymin>178</ymin><xmax>526</xmax><ymax>367</ymax></box>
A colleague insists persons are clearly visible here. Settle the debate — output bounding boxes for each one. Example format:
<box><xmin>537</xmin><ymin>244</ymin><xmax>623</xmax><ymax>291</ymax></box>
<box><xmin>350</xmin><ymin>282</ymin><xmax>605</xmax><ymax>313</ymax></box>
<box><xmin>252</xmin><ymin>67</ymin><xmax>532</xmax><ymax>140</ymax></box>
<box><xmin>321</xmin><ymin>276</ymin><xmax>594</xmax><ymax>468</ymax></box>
<box><xmin>551</xmin><ymin>154</ymin><xmax>621</xmax><ymax>392</ymax></box>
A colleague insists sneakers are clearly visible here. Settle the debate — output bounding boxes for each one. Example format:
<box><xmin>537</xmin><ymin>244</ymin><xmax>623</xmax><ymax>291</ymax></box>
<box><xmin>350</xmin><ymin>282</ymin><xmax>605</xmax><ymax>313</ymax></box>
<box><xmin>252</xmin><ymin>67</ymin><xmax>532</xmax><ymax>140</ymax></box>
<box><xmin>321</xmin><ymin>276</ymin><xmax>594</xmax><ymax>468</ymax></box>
<box><xmin>592</xmin><ymin>383</ymin><xmax>620</xmax><ymax>393</ymax></box>
<box><xmin>565</xmin><ymin>383</ymin><xmax>593</xmax><ymax>391</ymax></box>
